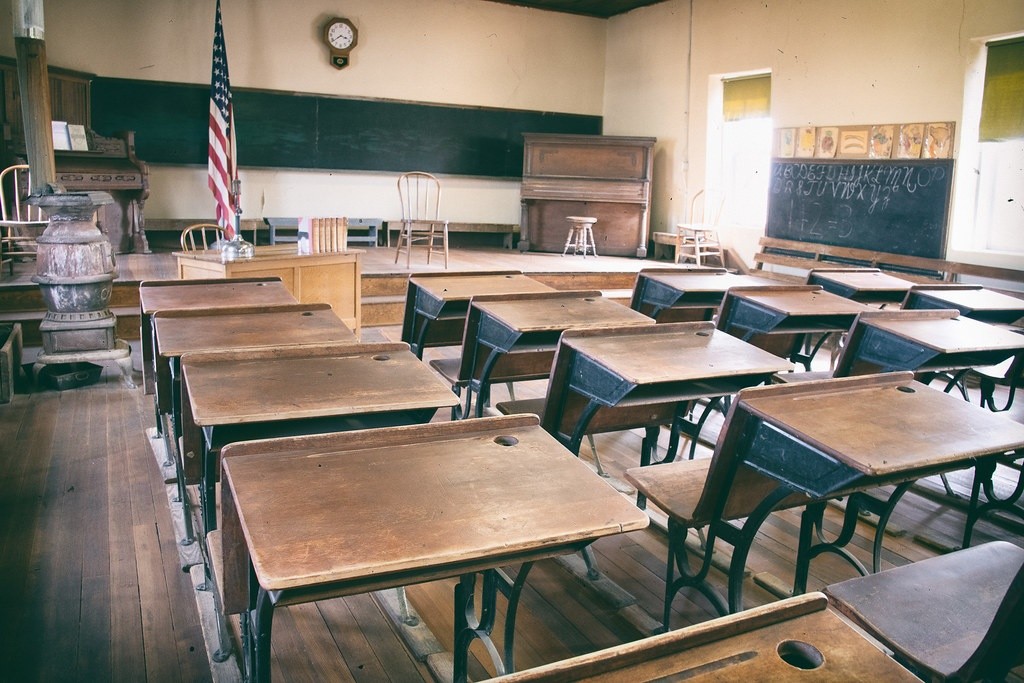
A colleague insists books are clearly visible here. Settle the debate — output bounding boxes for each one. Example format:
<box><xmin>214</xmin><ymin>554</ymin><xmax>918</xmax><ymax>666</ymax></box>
<box><xmin>297</xmin><ymin>217</ymin><xmax>347</xmax><ymax>255</ymax></box>
<box><xmin>51</xmin><ymin>121</ymin><xmax>88</xmax><ymax>151</ymax></box>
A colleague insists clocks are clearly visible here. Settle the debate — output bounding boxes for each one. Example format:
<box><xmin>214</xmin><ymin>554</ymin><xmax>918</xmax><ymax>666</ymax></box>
<box><xmin>324</xmin><ymin>17</ymin><xmax>359</xmax><ymax>69</ymax></box>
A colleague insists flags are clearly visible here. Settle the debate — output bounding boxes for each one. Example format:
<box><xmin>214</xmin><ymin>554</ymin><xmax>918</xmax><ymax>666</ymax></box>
<box><xmin>206</xmin><ymin>0</ymin><xmax>237</xmax><ymax>242</ymax></box>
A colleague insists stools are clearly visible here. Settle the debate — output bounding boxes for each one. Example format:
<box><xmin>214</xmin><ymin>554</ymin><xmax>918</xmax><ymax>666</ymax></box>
<box><xmin>560</xmin><ymin>215</ymin><xmax>599</xmax><ymax>259</ymax></box>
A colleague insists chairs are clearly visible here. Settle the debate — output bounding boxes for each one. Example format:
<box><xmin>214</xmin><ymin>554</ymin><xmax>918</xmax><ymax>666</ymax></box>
<box><xmin>672</xmin><ymin>188</ymin><xmax>727</xmax><ymax>269</ymax></box>
<box><xmin>0</xmin><ymin>164</ymin><xmax>51</xmax><ymax>277</ymax></box>
<box><xmin>180</xmin><ymin>223</ymin><xmax>233</xmax><ymax>253</ymax></box>
<box><xmin>394</xmin><ymin>172</ymin><xmax>448</xmax><ymax>269</ymax></box>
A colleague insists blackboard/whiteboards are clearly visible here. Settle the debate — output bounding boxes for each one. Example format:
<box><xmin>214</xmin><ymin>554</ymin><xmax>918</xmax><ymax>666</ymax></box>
<box><xmin>761</xmin><ymin>156</ymin><xmax>957</xmax><ymax>280</ymax></box>
<box><xmin>92</xmin><ymin>74</ymin><xmax>605</xmax><ymax>182</ymax></box>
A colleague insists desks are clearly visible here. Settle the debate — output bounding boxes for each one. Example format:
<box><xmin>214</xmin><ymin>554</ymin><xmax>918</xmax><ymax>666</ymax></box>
<box><xmin>134</xmin><ymin>268</ymin><xmax>1024</xmax><ymax>683</ymax></box>
<box><xmin>172</xmin><ymin>243</ymin><xmax>367</xmax><ymax>344</ymax></box>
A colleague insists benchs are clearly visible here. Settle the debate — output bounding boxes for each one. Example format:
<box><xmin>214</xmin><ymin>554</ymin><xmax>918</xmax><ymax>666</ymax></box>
<box><xmin>495</xmin><ymin>321</ymin><xmax>715</xmax><ymax>512</ymax></box>
<box><xmin>627</xmin><ymin>370</ymin><xmax>914</xmax><ymax>630</ymax></box>
<box><xmin>822</xmin><ymin>539</ymin><xmax>1024</xmax><ymax>683</ymax></box>
<box><xmin>774</xmin><ymin>309</ymin><xmax>960</xmax><ymax>396</ymax></box>
<box><xmin>709</xmin><ymin>285</ymin><xmax>824</xmax><ymax>374</ymax></box>
<box><xmin>900</xmin><ymin>283</ymin><xmax>983</xmax><ymax>313</ymax></box>
<box><xmin>429</xmin><ymin>292</ymin><xmax>601</xmax><ymax>401</ymax></box>
